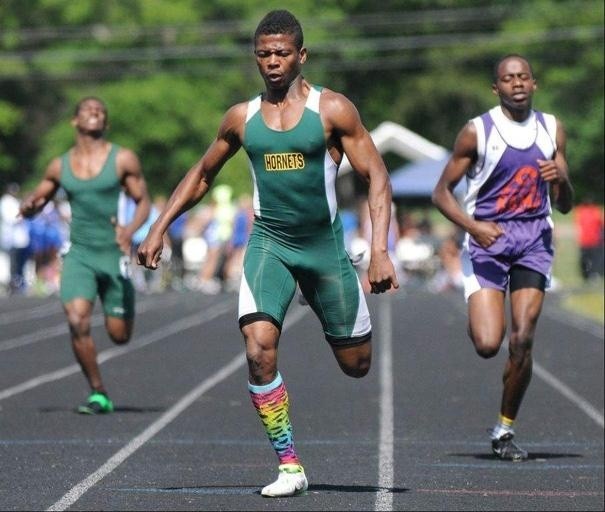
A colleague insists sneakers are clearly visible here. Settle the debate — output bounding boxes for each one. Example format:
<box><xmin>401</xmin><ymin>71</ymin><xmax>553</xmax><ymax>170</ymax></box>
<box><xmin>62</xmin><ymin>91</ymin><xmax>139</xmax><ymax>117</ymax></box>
<box><xmin>258</xmin><ymin>464</ymin><xmax>309</xmax><ymax>499</ymax></box>
<box><xmin>74</xmin><ymin>390</ymin><xmax>114</xmax><ymax>416</ymax></box>
<box><xmin>488</xmin><ymin>429</ymin><xmax>532</xmax><ymax>461</ymax></box>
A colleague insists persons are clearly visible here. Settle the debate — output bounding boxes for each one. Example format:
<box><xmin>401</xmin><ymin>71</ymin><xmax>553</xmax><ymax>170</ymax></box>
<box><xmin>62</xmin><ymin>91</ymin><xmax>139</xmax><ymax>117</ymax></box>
<box><xmin>137</xmin><ymin>7</ymin><xmax>401</xmax><ymax>499</ymax></box>
<box><xmin>18</xmin><ymin>91</ymin><xmax>154</xmax><ymax>418</ymax></box>
<box><xmin>430</xmin><ymin>54</ymin><xmax>575</xmax><ymax>462</ymax></box>
<box><xmin>1</xmin><ymin>181</ymin><xmax>604</xmax><ymax>306</ymax></box>
